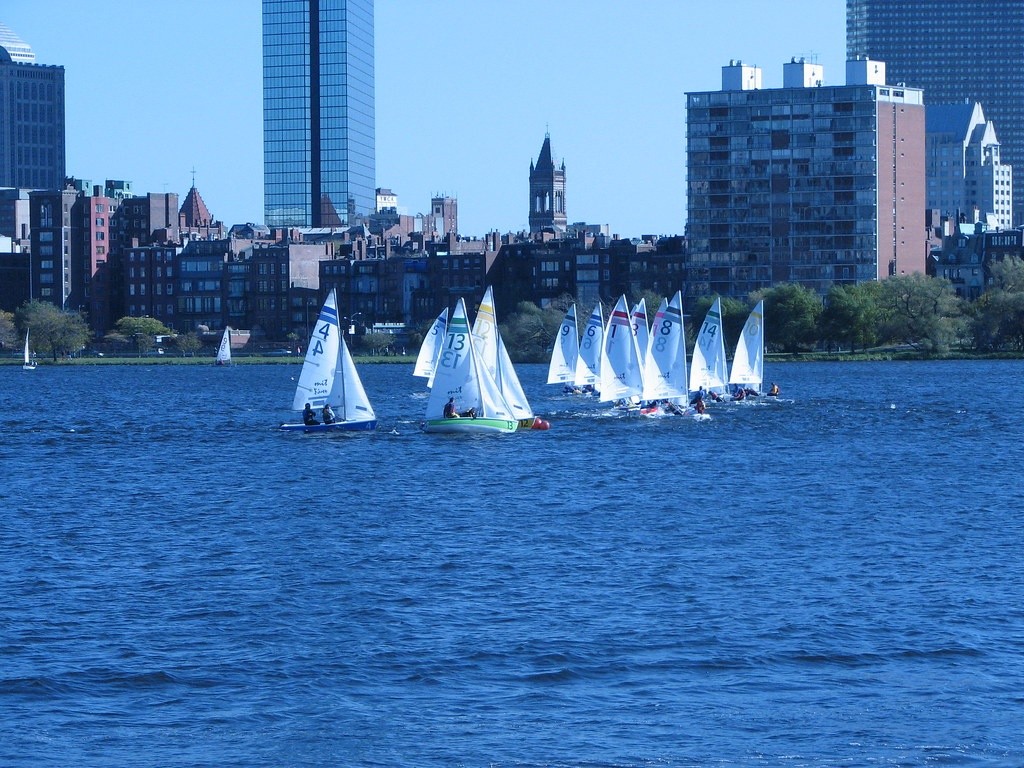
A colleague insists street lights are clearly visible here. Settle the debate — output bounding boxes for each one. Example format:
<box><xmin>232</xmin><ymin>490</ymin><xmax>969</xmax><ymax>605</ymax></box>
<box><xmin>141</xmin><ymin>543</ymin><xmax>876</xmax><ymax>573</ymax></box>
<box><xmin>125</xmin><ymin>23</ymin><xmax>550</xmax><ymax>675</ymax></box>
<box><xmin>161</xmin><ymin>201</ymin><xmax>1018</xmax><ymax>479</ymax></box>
<box><xmin>343</xmin><ymin>312</ymin><xmax>361</xmax><ymax>357</ymax></box>
<box><xmin>134</xmin><ymin>315</ymin><xmax>149</xmax><ymax>358</ymax></box>
<box><xmin>78</xmin><ymin>305</ymin><xmax>85</xmax><ymax>359</ymax></box>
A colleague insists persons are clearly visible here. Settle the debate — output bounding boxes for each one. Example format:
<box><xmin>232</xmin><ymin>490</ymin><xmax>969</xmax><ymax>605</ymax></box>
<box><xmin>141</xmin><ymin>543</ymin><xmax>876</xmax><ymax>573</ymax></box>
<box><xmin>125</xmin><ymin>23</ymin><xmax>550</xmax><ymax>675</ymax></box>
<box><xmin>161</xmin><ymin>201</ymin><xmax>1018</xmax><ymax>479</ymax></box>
<box><xmin>443</xmin><ymin>396</ymin><xmax>460</xmax><ymax>418</ymax></box>
<box><xmin>461</xmin><ymin>407</ymin><xmax>475</xmax><ymax>417</ymax></box>
<box><xmin>766</xmin><ymin>382</ymin><xmax>778</xmax><ymax>396</ymax></box>
<box><xmin>302</xmin><ymin>403</ymin><xmax>320</xmax><ymax>426</ymax></box>
<box><xmin>708</xmin><ymin>391</ymin><xmax>725</xmax><ymax>402</ymax></box>
<box><xmin>730</xmin><ymin>383</ymin><xmax>760</xmax><ymax>402</ymax></box>
<box><xmin>689</xmin><ymin>386</ymin><xmax>704</xmax><ymax>407</ymax></box>
<box><xmin>323</xmin><ymin>403</ymin><xmax>335</xmax><ymax>425</ymax></box>
<box><xmin>635</xmin><ymin>398</ymin><xmax>670</xmax><ymax>409</ymax></box>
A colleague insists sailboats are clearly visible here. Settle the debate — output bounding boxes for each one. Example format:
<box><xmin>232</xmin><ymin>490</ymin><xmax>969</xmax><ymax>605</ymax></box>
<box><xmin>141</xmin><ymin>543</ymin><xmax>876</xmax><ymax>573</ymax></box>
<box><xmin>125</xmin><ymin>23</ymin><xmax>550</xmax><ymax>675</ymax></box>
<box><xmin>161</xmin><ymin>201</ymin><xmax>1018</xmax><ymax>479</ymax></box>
<box><xmin>210</xmin><ymin>326</ymin><xmax>237</xmax><ymax>367</ymax></box>
<box><xmin>545</xmin><ymin>290</ymin><xmax>764</xmax><ymax>419</ymax></box>
<box><xmin>22</xmin><ymin>328</ymin><xmax>37</xmax><ymax>370</ymax></box>
<box><xmin>411</xmin><ymin>308</ymin><xmax>449</xmax><ymax>388</ymax></box>
<box><xmin>278</xmin><ymin>287</ymin><xmax>382</xmax><ymax>433</ymax></box>
<box><xmin>421</xmin><ymin>296</ymin><xmax>519</xmax><ymax>434</ymax></box>
<box><xmin>472</xmin><ymin>284</ymin><xmax>540</xmax><ymax>430</ymax></box>
